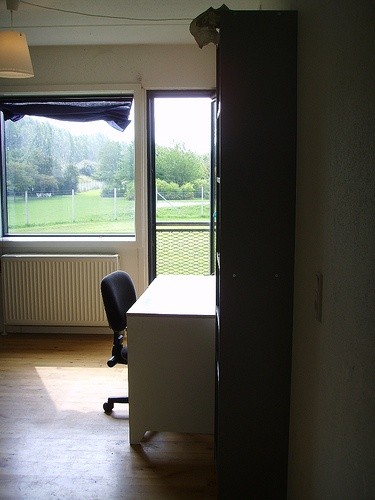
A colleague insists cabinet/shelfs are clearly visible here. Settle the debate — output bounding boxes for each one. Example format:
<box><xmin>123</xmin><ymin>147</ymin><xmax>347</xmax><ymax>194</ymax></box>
<box><xmin>210</xmin><ymin>9</ymin><xmax>300</xmax><ymax>500</ymax></box>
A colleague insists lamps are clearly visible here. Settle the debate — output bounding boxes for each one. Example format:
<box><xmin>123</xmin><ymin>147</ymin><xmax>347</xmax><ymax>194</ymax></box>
<box><xmin>0</xmin><ymin>0</ymin><xmax>34</xmax><ymax>78</ymax></box>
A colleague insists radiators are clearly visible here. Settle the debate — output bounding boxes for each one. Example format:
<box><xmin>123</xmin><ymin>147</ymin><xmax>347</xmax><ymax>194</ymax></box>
<box><xmin>0</xmin><ymin>254</ymin><xmax>119</xmax><ymax>326</ymax></box>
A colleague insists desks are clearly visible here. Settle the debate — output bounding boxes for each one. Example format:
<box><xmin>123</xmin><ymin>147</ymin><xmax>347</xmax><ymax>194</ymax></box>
<box><xmin>126</xmin><ymin>274</ymin><xmax>216</xmax><ymax>445</ymax></box>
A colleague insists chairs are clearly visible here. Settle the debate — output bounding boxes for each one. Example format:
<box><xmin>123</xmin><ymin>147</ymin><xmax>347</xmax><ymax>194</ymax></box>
<box><xmin>100</xmin><ymin>270</ymin><xmax>136</xmax><ymax>411</ymax></box>
